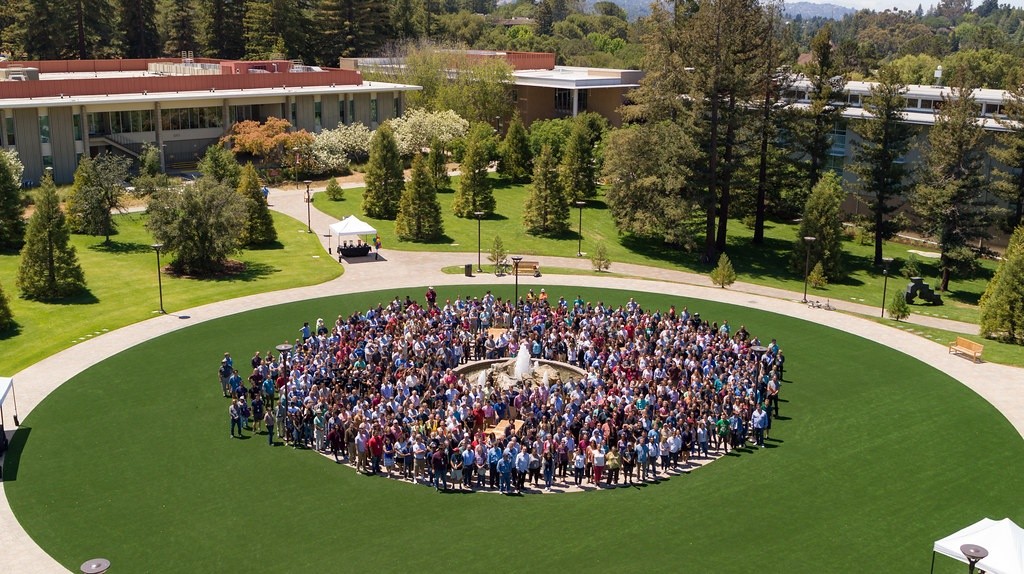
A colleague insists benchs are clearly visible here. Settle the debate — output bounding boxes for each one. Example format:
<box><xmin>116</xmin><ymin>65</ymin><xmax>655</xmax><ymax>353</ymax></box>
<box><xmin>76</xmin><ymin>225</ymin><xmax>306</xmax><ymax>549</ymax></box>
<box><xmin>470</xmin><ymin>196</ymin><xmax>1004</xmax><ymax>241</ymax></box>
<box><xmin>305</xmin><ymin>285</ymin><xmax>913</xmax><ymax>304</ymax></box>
<box><xmin>949</xmin><ymin>336</ymin><xmax>984</xmax><ymax>363</ymax></box>
<box><xmin>513</xmin><ymin>261</ymin><xmax>539</xmax><ymax>275</ymax></box>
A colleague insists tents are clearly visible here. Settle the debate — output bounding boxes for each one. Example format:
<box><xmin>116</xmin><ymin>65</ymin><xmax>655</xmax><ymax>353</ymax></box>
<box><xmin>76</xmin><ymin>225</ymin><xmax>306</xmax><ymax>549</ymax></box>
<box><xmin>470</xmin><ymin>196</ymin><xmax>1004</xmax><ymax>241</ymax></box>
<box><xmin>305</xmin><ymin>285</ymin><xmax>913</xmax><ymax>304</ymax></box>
<box><xmin>0</xmin><ymin>377</ymin><xmax>19</xmax><ymax>442</ymax></box>
<box><xmin>327</xmin><ymin>214</ymin><xmax>379</xmax><ymax>263</ymax></box>
<box><xmin>930</xmin><ymin>517</ymin><xmax>1024</xmax><ymax>574</ymax></box>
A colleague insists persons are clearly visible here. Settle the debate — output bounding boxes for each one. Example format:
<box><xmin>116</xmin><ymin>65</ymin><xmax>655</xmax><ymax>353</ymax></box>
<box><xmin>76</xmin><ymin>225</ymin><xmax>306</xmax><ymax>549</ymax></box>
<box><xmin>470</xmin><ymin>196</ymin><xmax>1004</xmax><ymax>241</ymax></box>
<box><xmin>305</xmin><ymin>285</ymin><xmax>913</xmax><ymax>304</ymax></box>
<box><xmin>218</xmin><ymin>360</ymin><xmax>234</xmax><ymax>397</ymax></box>
<box><xmin>313</xmin><ymin>409</ymin><xmax>328</xmax><ymax>453</ymax></box>
<box><xmin>262</xmin><ymin>186</ymin><xmax>271</xmax><ymax>199</ymax></box>
<box><xmin>234</xmin><ymin>380</ymin><xmax>248</xmax><ymax>402</ymax></box>
<box><xmin>237</xmin><ymin>396</ymin><xmax>250</xmax><ymax>429</ymax></box>
<box><xmin>248</xmin><ymin>287</ymin><xmax>784</xmax><ymax>495</ymax></box>
<box><xmin>354</xmin><ymin>428</ymin><xmax>368</xmax><ymax>475</ymax></box>
<box><xmin>251</xmin><ymin>394</ymin><xmax>264</xmax><ymax>431</ymax></box>
<box><xmin>228</xmin><ymin>399</ymin><xmax>243</xmax><ymax>438</ymax></box>
<box><xmin>299</xmin><ymin>321</ymin><xmax>310</xmax><ymax>344</ymax></box>
<box><xmin>264</xmin><ymin>407</ymin><xmax>276</xmax><ymax>447</ymax></box>
<box><xmin>273</xmin><ymin>400</ymin><xmax>286</xmax><ymax>438</ymax></box>
<box><xmin>251</xmin><ymin>351</ymin><xmax>262</xmax><ymax>368</ymax></box>
<box><xmin>228</xmin><ymin>370</ymin><xmax>243</xmax><ymax>399</ymax></box>
<box><xmin>262</xmin><ymin>375</ymin><xmax>275</xmax><ymax>412</ymax></box>
<box><xmin>222</xmin><ymin>352</ymin><xmax>233</xmax><ymax>389</ymax></box>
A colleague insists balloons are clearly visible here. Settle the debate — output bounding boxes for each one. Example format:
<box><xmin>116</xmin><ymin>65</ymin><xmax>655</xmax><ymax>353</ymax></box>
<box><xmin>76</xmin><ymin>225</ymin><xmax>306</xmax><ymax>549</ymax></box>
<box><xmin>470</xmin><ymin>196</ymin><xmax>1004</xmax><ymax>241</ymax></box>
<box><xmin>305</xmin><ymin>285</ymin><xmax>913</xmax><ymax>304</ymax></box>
<box><xmin>373</xmin><ymin>236</ymin><xmax>382</xmax><ymax>249</ymax></box>
